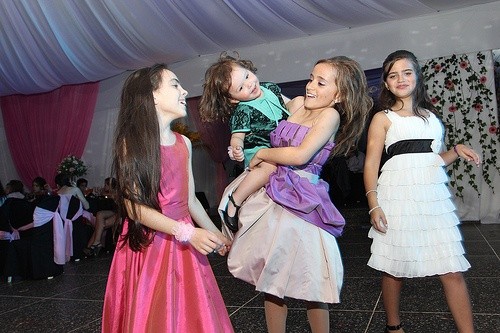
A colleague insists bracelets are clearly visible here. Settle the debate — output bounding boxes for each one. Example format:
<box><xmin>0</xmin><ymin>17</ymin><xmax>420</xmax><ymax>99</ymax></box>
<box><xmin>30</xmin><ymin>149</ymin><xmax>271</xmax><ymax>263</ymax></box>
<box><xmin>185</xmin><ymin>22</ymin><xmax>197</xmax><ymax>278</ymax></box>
<box><xmin>171</xmin><ymin>221</ymin><xmax>194</xmax><ymax>242</ymax></box>
<box><xmin>365</xmin><ymin>189</ymin><xmax>378</xmax><ymax>197</ymax></box>
<box><xmin>368</xmin><ymin>205</ymin><xmax>381</xmax><ymax>215</ymax></box>
<box><xmin>228</xmin><ymin>145</ymin><xmax>244</xmax><ymax>161</ymax></box>
<box><xmin>453</xmin><ymin>144</ymin><xmax>460</xmax><ymax>158</ymax></box>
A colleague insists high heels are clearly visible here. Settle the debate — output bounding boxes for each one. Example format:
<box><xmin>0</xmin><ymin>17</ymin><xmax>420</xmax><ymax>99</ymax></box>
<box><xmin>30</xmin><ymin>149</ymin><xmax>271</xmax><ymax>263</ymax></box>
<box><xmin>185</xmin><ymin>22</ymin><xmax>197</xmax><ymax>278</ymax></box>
<box><xmin>83</xmin><ymin>243</ymin><xmax>102</xmax><ymax>255</ymax></box>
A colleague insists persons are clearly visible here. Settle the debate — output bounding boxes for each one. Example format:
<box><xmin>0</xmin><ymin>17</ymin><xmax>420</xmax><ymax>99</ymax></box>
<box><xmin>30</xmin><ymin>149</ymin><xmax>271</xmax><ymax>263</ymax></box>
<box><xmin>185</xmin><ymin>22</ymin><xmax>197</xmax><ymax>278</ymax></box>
<box><xmin>28</xmin><ymin>177</ymin><xmax>54</xmax><ymax>204</ymax></box>
<box><xmin>0</xmin><ymin>179</ymin><xmax>33</xmax><ymax>236</ymax></box>
<box><xmin>218</xmin><ymin>56</ymin><xmax>373</xmax><ymax>333</ymax></box>
<box><xmin>83</xmin><ymin>176</ymin><xmax>125</xmax><ymax>257</ymax></box>
<box><xmin>364</xmin><ymin>50</ymin><xmax>484</xmax><ymax>333</ymax></box>
<box><xmin>101</xmin><ymin>62</ymin><xmax>234</xmax><ymax>333</ymax></box>
<box><xmin>343</xmin><ymin>144</ymin><xmax>366</xmax><ymax>207</ymax></box>
<box><xmin>199</xmin><ymin>58</ymin><xmax>292</xmax><ymax>233</ymax></box>
<box><xmin>54</xmin><ymin>173</ymin><xmax>95</xmax><ymax>261</ymax></box>
<box><xmin>77</xmin><ymin>177</ymin><xmax>88</xmax><ymax>196</ymax></box>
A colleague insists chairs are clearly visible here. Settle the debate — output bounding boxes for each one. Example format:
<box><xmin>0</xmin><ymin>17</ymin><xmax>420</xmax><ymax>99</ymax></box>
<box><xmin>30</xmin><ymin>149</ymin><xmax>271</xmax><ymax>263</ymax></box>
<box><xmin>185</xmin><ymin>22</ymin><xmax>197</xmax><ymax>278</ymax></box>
<box><xmin>0</xmin><ymin>198</ymin><xmax>34</xmax><ymax>289</ymax></box>
<box><xmin>58</xmin><ymin>195</ymin><xmax>94</xmax><ymax>262</ymax></box>
<box><xmin>32</xmin><ymin>195</ymin><xmax>65</xmax><ymax>280</ymax></box>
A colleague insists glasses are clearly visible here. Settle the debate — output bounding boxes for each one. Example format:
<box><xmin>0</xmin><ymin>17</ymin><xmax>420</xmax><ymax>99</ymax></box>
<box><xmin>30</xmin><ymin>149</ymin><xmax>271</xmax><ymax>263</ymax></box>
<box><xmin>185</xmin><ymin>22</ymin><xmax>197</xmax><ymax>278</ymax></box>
<box><xmin>80</xmin><ymin>185</ymin><xmax>87</xmax><ymax>187</ymax></box>
<box><xmin>32</xmin><ymin>184</ymin><xmax>40</xmax><ymax>187</ymax></box>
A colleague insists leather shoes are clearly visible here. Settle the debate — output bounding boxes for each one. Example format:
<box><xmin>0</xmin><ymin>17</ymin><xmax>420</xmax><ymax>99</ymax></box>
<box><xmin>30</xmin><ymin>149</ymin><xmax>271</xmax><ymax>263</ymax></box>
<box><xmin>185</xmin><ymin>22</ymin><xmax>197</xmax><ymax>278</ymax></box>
<box><xmin>224</xmin><ymin>193</ymin><xmax>240</xmax><ymax>231</ymax></box>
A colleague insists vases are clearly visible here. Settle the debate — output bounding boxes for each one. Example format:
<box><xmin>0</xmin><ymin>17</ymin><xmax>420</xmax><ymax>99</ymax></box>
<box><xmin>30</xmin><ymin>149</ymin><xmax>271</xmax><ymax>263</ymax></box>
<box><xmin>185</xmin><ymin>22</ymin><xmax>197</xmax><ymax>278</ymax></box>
<box><xmin>70</xmin><ymin>176</ymin><xmax>77</xmax><ymax>188</ymax></box>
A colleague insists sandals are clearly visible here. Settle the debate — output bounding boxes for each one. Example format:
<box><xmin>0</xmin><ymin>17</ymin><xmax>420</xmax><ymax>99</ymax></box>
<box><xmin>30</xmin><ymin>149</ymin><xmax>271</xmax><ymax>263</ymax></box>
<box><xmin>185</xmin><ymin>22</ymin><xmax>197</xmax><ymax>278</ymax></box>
<box><xmin>384</xmin><ymin>321</ymin><xmax>407</xmax><ymax>333</ymax></box>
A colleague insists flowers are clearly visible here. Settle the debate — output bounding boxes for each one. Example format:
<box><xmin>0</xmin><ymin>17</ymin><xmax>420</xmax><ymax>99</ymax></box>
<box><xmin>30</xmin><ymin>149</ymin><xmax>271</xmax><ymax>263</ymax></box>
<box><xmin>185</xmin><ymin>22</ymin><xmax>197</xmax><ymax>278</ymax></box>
<box><xmin>56</xmin><ymin>153</ymin><xmax>88</xmax><ymax>177</ymax></box>
<box><xmin>421</xmin><ymin>51</ymin><xmax>500</xmax><ymax>203</ymax></box>
<box><xmin>170</xmin><ymin>121</ymin><xmax>203</xmax><ymax>142</ymax></box>
